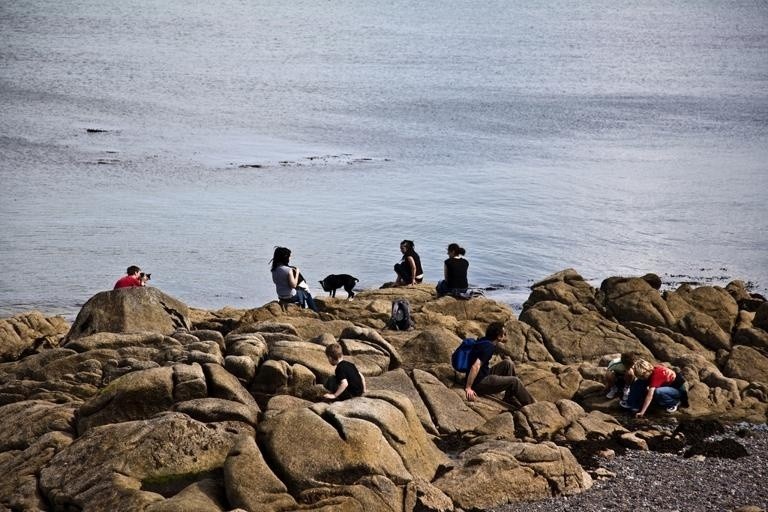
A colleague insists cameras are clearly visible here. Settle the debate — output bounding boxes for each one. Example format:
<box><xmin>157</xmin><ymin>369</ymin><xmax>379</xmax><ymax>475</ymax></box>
<box><xmin>140</xmin><ymin>273</ymin><xmax>151</xmax><ymax>280</ymax></box>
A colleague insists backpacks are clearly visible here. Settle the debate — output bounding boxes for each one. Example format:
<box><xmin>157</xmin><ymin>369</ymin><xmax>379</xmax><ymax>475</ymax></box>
<box><xmin>390</xmin><ymin>298</ymin><xmax>412</xmax><ymax>332</ymax></box>
<box><xmin>448</xmin><ymin>337</ymin><xmax>496</xmax><ymax>374</ymax></box>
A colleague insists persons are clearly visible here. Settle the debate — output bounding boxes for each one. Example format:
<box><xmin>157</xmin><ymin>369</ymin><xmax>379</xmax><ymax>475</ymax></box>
<box><xmin>322</xmin><ymin>342</ymin><xmax>368</xmax><ymax>403</ymax></box>
<box><xmin>268</xmin><ymin>244</ymin><xmax>319</xmax><ymax>313</ymax></box>
<box><xmin>114</xmin><ymin>264</ymin><xmax>149</xmax><ymax>290</ymax></box>
<box><xmin>604</xmin><ymin>348</ymin><xmax>637</xmax><ymax>408</ymax></box>
<box><xmin>435</xmin><ymin>242</ymin><xmax>468</xmax><ymax>296</ymax></box>
<box><xmin>462</xmin><ymin>320</ymin><xmax>535</xmax><ymax>410</ymax></box>
<box><xmin>390</xmin><ymin>239</ymin><xmax>424</xmax><ymax>289</ymax></box>
<box><xmin>627</xmin><ymin>357</ymin><xmax>690</xmax><ymax>418</ymax></box>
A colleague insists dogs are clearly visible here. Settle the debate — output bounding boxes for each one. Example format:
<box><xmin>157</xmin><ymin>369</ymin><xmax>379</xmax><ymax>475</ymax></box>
<box><xmin>316</xmin><ymin>274</ymin><xmax>360</xmax><ymax>299</ymax></box>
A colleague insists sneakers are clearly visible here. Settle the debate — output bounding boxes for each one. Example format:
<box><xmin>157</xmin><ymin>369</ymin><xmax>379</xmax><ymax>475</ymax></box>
<box><xmin>605</xmin><ymin>384</ymin><xmax>640</xmax><ymax>413</ymax></box>
<box><xmin>665</xmin><ymin>399</ymin><xmax>682</xmax><ymax>413</ymax></box>
